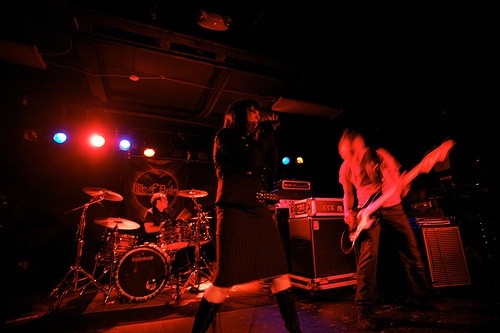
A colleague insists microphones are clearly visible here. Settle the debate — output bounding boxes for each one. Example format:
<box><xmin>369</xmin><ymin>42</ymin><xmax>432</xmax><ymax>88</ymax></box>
<box><xmin>258</xmin><ymin>120</ymin><xmax>279</xmax><ymax>126</ymax></box>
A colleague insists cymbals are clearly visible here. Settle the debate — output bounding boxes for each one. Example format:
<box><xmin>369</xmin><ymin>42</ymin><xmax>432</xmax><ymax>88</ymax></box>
<box><xmin>82</xmin><ymin>186</ymin><xmax>123</xmax><ymax>201</ymax></box>
<box><xmin>177</xmin><ymin>189</ymin><xmax>208</xmax><ymax>198</ymax></box>
<box><xmin>94</xmin><ymin>218</ymin><xmax>140</xmax><ymax>230</ymax></box>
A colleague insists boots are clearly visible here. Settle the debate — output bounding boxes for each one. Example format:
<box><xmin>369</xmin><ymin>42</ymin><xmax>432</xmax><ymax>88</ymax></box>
<box><xmin>273</xmin><ymin>286</ymin><xmax>302</xmax><ymax>333</ymax></box>
<box><xmin>191</xmin><ymin>296</ymin><xmax>222</xmax><ymax>333</ymax></box>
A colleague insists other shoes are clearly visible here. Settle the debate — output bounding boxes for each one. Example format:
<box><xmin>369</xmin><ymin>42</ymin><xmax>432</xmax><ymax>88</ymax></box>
<box><xmin>410</xmin><ymin>301</ymin><xmax>437</xmax><ymax>313</ymax></box>
<box><xmin>356</xmin><ymin>309</ymin><xmax>377</xmax><ymax>330</ymax></box>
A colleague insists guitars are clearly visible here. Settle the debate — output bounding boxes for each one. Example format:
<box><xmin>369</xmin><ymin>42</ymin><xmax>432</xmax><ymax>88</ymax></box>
<box><xmin>341</xmin><ymin>140</ymin><xmax>452</xmax><ymax>254</ymax></box>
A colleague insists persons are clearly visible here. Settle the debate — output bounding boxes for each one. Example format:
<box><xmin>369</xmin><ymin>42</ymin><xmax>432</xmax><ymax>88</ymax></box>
<box><xmin>339</xmin><ymin>130</ymin><xmax>435</xmax><ymax>324</ymax></box>
<box><xmin>192</xmin><ymin>99</ymin><xmax>303</xmax><ymax>333</ymax></box>
<box><xmin>143</xmin><ymin>193</ymin><xmax>171</xmax><ymax>241</ymax></box>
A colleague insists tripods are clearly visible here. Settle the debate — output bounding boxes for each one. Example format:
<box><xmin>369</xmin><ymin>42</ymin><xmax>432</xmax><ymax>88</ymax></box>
<box><xmin>179</xmin><ymin>197</ymin><xmax>213</xmax><ymax>294</ymax></box>
<box><xmin>46</xmin><ymin>197</ymin><xmax>118</xmax><ymax>309</ymax></box>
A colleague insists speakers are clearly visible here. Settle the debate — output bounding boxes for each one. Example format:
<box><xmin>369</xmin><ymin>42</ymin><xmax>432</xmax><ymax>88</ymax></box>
<box><xmin>410</xmin><ymin>225</ymin><xmax>471</xmax><ymax>288</ymax></box>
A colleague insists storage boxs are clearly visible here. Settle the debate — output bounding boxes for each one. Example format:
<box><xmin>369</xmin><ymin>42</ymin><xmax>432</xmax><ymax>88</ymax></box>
<box><xmin>288</xmin><ymin>197</ymin><xmax>344</xmax><ymax>218</ymax></box>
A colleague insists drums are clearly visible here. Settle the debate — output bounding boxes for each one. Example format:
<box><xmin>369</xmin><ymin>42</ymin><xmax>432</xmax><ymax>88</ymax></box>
<box><xmin>158</xmin><ymin>219</ymin><xmax>190</xmax><ymax>250</ymax></box>
<box><xmin>114</xmin><ymin>244</ymin><xmax>170</xmax><ymax>302</ymax></box>
<box><xmin>101</xmin><ymin>233</ymin><xmax>139</xmax><ymax>261</ymax></box>
<box><xmin>186</xmin><ymin>216</ymin><xmax>214</xmax><ymax>246</ymax></box>
